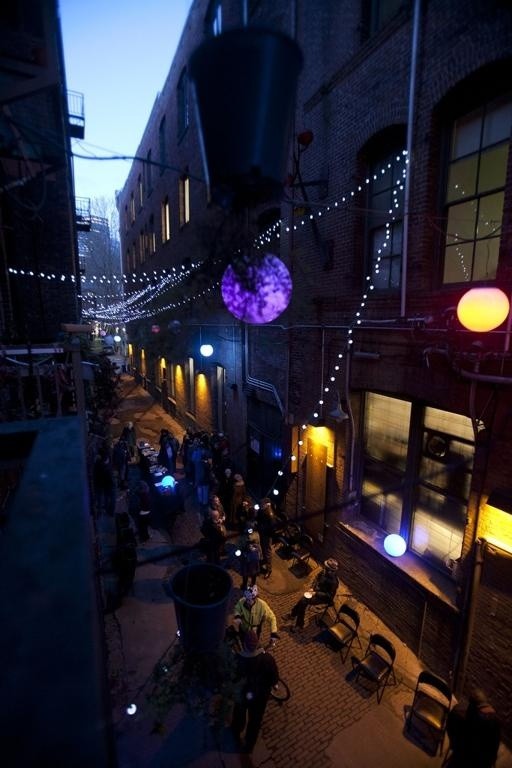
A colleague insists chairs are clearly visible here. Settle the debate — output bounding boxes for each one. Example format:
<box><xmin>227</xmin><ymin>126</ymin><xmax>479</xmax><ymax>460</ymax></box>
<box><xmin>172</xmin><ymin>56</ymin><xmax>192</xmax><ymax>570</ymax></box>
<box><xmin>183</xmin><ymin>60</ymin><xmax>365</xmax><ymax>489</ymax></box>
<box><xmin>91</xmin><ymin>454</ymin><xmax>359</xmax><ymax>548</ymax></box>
<box><xmin>355</xmin><ymin>633</ymin><xmax>397</xmax><ymax>704</ymax></box>
<box><xmin>408</xmin><ymin>671</ymin><xmax>452</xmax><ymax>756</ymax></box>
<box><xmin>291</xmin><ymin>534</ymin><xmax>315</xmax><ymax>575</ymax></box>
<box><xmin>324</xmin><ymin>603</ymin><xmax>363</xmax><ymax>664</ymax></box>
<box><xmin>308</xmin><ymin>575</ymin><xmax>339</xmax><ymax>624</ymax></box>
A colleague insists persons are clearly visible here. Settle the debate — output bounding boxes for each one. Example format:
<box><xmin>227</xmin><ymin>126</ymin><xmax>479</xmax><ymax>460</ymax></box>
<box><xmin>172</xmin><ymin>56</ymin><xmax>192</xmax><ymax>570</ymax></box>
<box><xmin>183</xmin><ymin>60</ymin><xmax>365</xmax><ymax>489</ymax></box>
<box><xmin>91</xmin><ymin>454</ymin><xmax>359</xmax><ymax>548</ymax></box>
<box><xmin>232</xmin><ymin>582</ymin><xmax>278</xmax><ymax>654</ymax></box>
<box><xmin>444</xmin><ymin>689</ymin><xmax>503</xmax><ymax>767</ymax></box>
<box><xmin>281</xmin><ymin>557</ymin><xmax>339</xmax><ymax>635</ymax></box>
<box><xmin>227</xmin><ymin>633</ymin><xmax>279</xmax><ymax>755</ymax></box>
<box><xmin>92</xmin><ymin>418</ymin><xmax>279</xmax><ymax>600</ymax></box>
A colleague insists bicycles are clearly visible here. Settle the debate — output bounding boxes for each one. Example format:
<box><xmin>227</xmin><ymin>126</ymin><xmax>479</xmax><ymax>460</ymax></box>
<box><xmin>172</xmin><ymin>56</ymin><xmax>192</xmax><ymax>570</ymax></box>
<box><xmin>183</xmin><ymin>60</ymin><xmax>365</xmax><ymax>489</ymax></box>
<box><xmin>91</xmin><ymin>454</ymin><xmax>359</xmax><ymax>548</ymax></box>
<box><xmin>225</xmin><ymin>625</ymin><xmax>290</xmax><ymax>702</ymax></box>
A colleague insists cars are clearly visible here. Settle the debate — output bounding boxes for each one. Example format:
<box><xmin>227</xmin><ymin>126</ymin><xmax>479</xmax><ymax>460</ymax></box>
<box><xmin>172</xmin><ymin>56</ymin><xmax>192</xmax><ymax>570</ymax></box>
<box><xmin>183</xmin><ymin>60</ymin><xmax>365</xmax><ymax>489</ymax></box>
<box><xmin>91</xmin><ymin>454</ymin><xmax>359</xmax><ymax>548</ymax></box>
<box><xmin>103</xmin><ymin>345</ymin><xmax>115</xmax><ymax>355</ymax></box>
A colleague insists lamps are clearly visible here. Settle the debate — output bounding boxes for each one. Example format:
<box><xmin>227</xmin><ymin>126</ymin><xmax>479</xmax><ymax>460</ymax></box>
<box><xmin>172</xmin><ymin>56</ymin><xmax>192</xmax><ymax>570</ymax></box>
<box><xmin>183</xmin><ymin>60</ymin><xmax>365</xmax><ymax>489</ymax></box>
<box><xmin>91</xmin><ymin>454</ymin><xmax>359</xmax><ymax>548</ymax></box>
<box><xmin>384</xmin><ymin>534</ymin><xmax>407</xmax><ymax>557</ymax></box>
<box><xmin>457</xmin><ymin>220</ymin><xmax>510</xmax><ymax>333</ymax></box>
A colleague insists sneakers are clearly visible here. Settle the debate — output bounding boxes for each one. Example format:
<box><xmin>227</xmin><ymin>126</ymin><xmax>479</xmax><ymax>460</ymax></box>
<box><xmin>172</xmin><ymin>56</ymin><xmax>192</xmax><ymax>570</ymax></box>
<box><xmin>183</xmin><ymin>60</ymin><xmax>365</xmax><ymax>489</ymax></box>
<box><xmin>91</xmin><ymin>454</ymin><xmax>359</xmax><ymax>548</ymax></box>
<box><xmin>281</xmin><ymin>611</ymin><xmax>303</xmax><ymax>634</ymax></box>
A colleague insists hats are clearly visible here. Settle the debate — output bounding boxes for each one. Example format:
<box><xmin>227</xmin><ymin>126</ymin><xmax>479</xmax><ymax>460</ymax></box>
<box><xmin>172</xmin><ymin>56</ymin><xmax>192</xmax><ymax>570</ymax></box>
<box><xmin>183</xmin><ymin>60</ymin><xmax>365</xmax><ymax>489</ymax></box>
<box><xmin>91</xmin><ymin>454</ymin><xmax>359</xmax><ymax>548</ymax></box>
<box><xmin>324</xmin><ymin>558</ymin><xmax>340</xmax><ymax>572</ymax></box>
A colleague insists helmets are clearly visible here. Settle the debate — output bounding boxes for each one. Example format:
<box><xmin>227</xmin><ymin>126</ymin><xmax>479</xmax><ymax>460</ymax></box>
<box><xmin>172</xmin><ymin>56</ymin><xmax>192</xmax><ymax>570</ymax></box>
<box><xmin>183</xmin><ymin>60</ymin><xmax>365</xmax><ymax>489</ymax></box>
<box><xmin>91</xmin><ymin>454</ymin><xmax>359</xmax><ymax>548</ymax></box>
<box><xmin>243</xmin><ymin>583</ymin><xmax>259</xmax><ymax>600</ymax></box>
